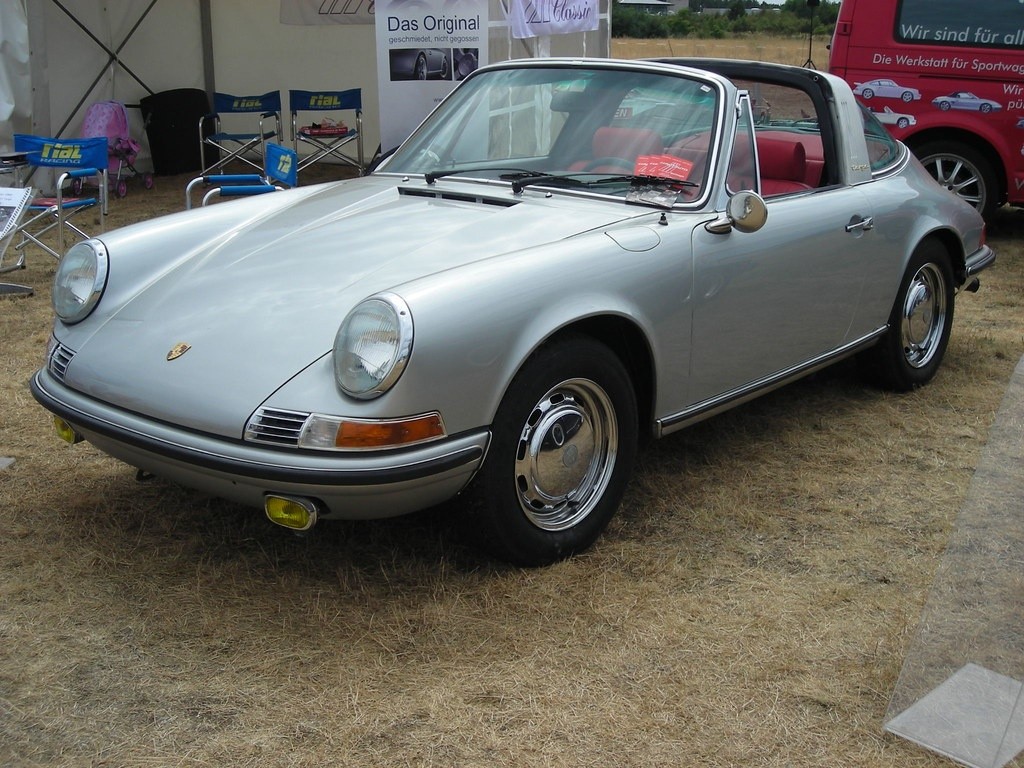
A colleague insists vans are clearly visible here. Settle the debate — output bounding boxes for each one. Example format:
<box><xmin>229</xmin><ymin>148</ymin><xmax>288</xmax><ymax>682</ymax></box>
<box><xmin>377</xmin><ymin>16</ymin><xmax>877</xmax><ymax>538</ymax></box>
<box><xmin>827</xmin><ymin>0</ymin><xmax>1024</xmax><ymax>228</ymax></box>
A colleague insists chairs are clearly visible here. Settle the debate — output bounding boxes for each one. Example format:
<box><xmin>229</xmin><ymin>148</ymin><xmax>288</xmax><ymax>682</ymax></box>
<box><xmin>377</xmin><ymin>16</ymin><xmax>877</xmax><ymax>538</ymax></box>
<box><xmin>570</xmin><ymin>128</ymin><xmax>663</xmax><ymax>173</ymax></box>
<box><xmin>684</xmin><ymin>135</ymin><xmax>813</xmax><ymax>198</ymax></box>
<box><xmin>199</xmin><ymin>90</ymin><xmax>289</xmax><ymax>172</ymax></box>
<box><xmin>0</xmin><ymin>133</ymin><xmax>110</xmax><ymax>270</ymax></box>
<box><xmin>184</xmin><ymin>142</ymin><xmax>298</xmax><ymax>209</ymax></box>
<box><xmin>0</xmin><ymin>186</ymin><xmax>40</xmax><ymax>297</ymax></box>
<box><xmin>286</xmin><ymin>87</ymin><xmax>366</xmax><ymax>177</ymax></box>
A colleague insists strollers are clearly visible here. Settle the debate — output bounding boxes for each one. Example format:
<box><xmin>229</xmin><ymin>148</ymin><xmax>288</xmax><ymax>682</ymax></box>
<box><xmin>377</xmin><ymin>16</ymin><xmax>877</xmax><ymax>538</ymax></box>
<box><xmin>68</xmin><ymin>100</ymin><xmax>154</xmax><ymax>198</ymax></box>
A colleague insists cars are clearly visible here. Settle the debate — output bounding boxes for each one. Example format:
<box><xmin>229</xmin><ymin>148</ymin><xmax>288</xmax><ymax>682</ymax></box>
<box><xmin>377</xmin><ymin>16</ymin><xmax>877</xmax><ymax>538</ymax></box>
<box><xmin>27</xmin><ymin>53</ymin><xmax>996</xmax><ymax>568</ymax></box>
<box><xmin>930</xmin><ymin>91</ymin><xmax>1003</xmax><ymax>114</ymax></box>
<box><xmin>864</xmin><ymin>105</ymin><xmax>918</xmax><ymax>129</ymax></box>
<box><xmin>851</xmin><ymin>78</ymin><xmax>922</xmax><ymax>102</ymax></box>
